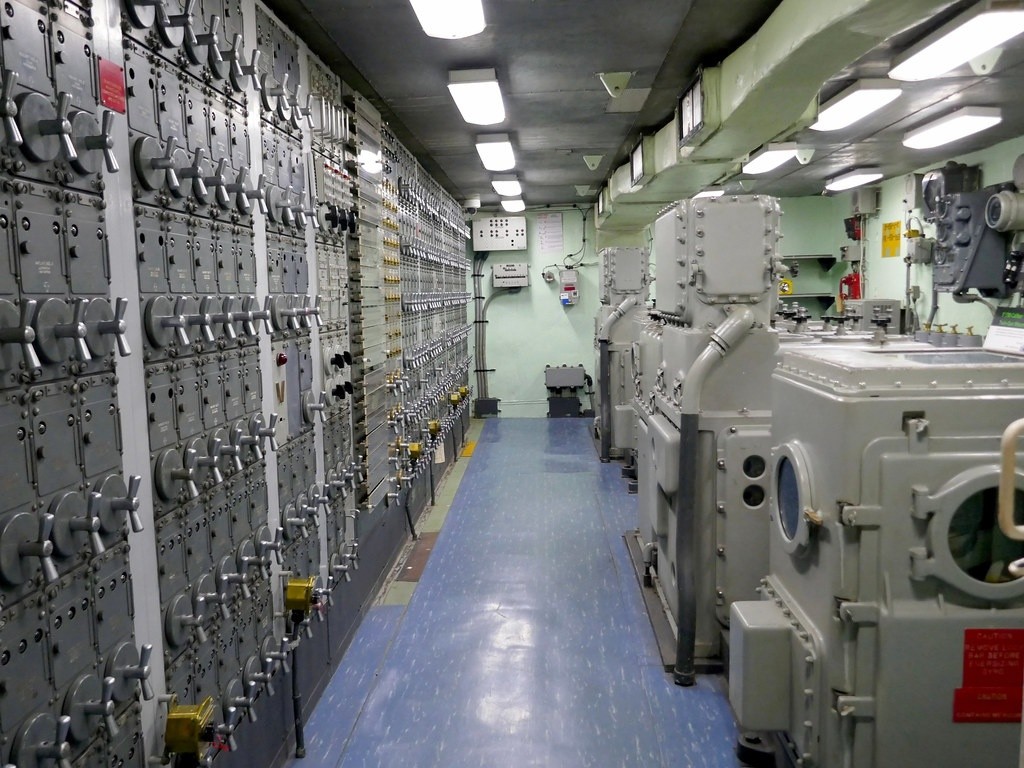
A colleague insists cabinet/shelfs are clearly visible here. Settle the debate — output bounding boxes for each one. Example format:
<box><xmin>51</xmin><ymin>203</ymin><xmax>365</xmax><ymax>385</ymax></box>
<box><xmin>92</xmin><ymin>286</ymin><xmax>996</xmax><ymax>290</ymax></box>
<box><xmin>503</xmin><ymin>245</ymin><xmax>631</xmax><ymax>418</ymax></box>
<box><xmin>780</xmin><ymin>253</ymin><xmax>837</xmax><ymax>310</ymax></box>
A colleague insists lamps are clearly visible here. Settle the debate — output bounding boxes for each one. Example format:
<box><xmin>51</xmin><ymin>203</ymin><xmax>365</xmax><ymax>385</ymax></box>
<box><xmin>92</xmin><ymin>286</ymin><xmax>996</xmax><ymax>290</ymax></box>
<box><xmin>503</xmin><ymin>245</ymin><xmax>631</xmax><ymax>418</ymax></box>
<box><xmin>475</xmin><ymin>133</ymin><xmax>515</xmax><ymax>172</ymax></box>
<box><xmin>885</xmin><ymin>0</ymin><xmax>1024</xmax><ymax>82</ymax></box>
<box><xmin>446</xmin><ymin>67</ymin><xmax>506</xmax><ymax>126</ymax></box>
<box><xmin>808</xmin><ymin>78</ymin><xmax>903</xmax><ymax>132</ymax></box>
<box><xmin>742</xmin><ymin>141</ymin><xmax>798</xmax><ymax>175</ymax></box>
<box><xmin>693</xmin><ymin>186</ymin><xmax>725</xmax><ymax>198</ymax></box>
<box><xmin>501</xmin><ymin>195</ymin><xmax>526</xmax><ymax>213</ymax></box>
<box><xmin>491</xmin><ymin>174</ymin><xmax>522</xmax><ymax>196</ymax></box>
<box><xmin>902</xmin><ymin>105</ymin><xmax>1004</xmax><ymax>150</ymax></box>
<box><xmin>825</xmin><ymin>167</ymin><xmax>884</xmax><ymax>191</ymax></box>
